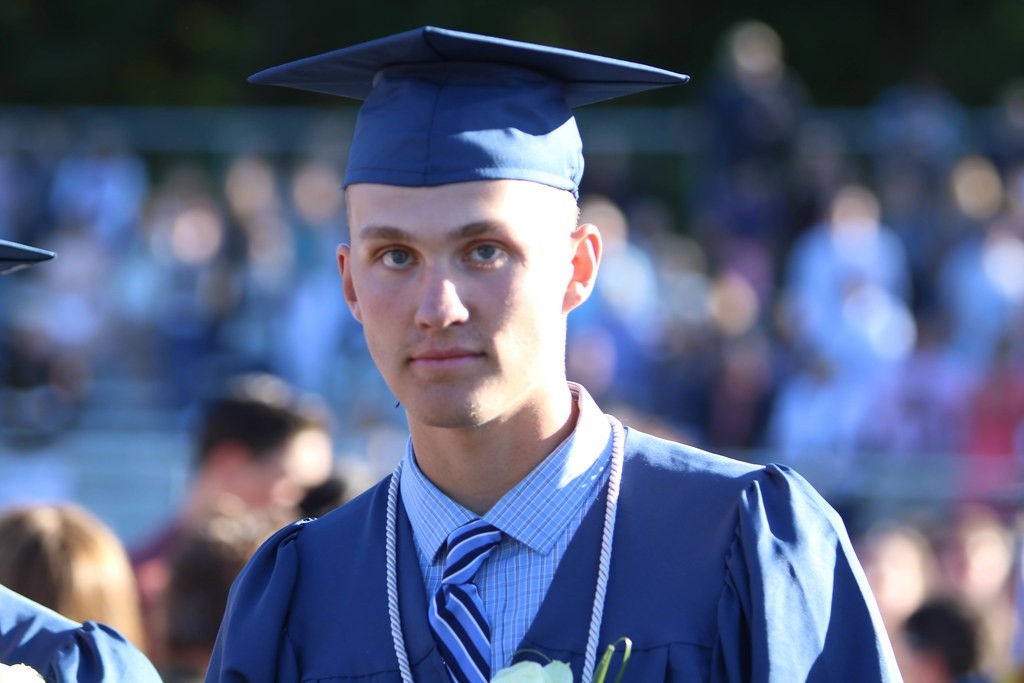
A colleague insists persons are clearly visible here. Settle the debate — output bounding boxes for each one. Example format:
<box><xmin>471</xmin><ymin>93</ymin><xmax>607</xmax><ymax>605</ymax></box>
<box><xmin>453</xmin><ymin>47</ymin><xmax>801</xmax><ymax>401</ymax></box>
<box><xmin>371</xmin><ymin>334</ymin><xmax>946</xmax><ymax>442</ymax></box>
<box><xmin>205</xmin><ymin>27</ymin><xmax>900</xmax><ymax>683</ymax></box>
<box><xmin>0</xmin><ymin>21</ymin><xmax>1024</xmax><ymax>683</ymax></box>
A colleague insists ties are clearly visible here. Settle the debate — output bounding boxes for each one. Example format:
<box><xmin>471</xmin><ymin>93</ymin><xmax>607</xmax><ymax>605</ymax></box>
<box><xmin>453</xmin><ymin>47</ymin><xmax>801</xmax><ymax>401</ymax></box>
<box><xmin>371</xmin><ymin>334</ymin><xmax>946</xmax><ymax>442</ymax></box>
<box><xmin>425</xmin><ymin>519</ymin><xmax>507</xmax><ymax>682</ymax></box>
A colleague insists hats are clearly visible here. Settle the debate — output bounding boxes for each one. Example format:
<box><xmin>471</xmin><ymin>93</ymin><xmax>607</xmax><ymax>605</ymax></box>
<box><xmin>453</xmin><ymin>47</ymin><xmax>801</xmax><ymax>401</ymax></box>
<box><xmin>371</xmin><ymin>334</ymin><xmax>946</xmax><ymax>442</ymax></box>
<box><xmin>245</xmin><ymin>24</ymin><xmax>693</xmax><ymax>198</ymax></box>
<box><xmin>3</xmin><ymin>235</ymin><xmax>51</xmax><ymax>271</ymax></box>
